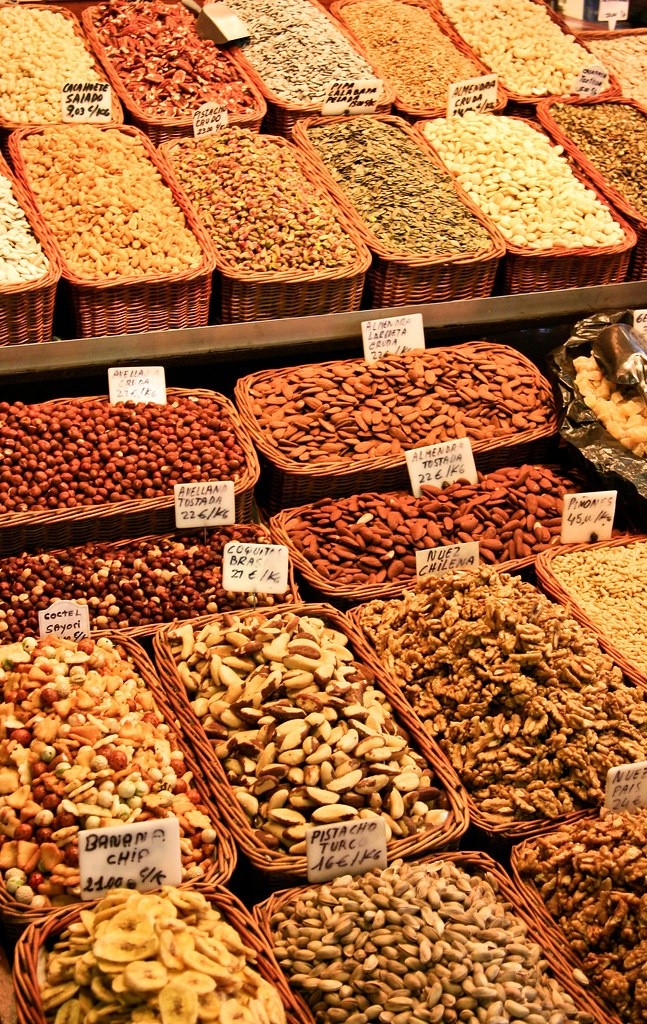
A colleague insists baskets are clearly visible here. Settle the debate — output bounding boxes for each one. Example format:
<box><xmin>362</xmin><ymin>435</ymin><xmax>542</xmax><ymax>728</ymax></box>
<box><xmin>12</xmin><ymin>879</ymin><xmax>317</xmax><ymax>1024</ymax></box>
<box><xmin>1</xmin><ymin>0</ymin><xmax>647</xmax><ymax>344</ymax></box>
<box><xmin>234</xmin><ymin>340</ymin><xmax>564</xmax><ymax>512</ymax></box>
<box><xmin>346</xmin><ymin>584</ymin><xmax>602</xmax><ymax>852</ymax></box>
<box><xmin>0</xmin><ymin>388</ymin><xmax>260</xmax><ymax>563</ymax></box>
<box><xmin>270</xmin><ymin>463</ymin><xmax>598</xmax><ymax>602</ymax></box>
<box><xmin>154</xmin><ymin>601</ymin><xmax>471</xmax><ymax>879</ymax></box>
<box><xmin>537</xmin><ymin>536</ymin><xmax>646</xmax><ymax>692</ymax></box>
<box><xmin>252</xmin><ymin>850</ymin><xmax>613</xmax><ymax>1024</ymax></box>
<box><xmin>511</xmin><ymin>813</ymin><xmax>625</xmax><ymax>1024</ymax></box>
<box><xmin>4</xmin><ymin>523</ymin><xmax>303</xmax><ymax>656</ymax></box>
<box><xmin>1</xmin><ymin>631</ymin><xmax>236</xmax><ymax>928</ymax></box>
